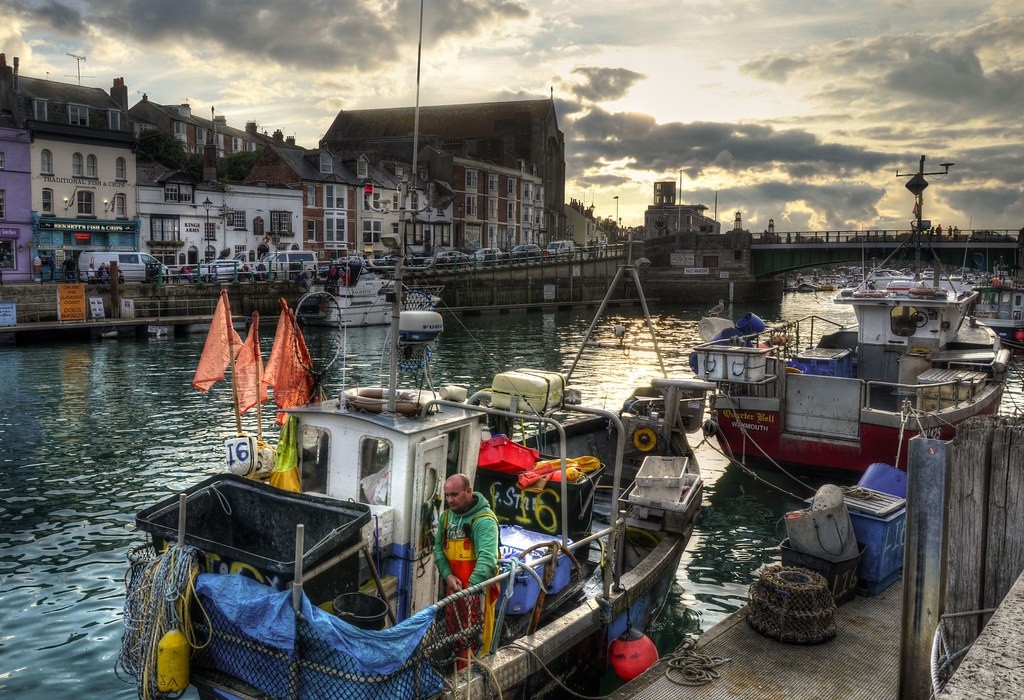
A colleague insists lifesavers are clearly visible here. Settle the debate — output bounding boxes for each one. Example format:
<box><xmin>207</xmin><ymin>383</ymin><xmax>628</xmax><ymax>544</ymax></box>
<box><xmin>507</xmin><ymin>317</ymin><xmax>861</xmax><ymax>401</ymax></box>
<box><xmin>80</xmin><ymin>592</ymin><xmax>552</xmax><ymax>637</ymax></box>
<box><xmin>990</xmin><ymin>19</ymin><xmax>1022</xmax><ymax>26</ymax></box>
<box><xmin>342</xmin><ymin>272</ymin><xmax>350</xmax><ymax>282</ymax></box>
<box><xmin>338</xmin><ymin>386</ymin><xmax>424</xmax><ymax>413</ymax></box>
<box><xmin>331</xmin><ymin>268</ymin><xmax>336</xmax><ymax>276</ymax></box>
<box><xmin>909</xmin><ymin>287</ymin><xmax>936</xmax><ymax>299</ymax></box>
<box><xmin>853</xmin><ymin>290</ymin><xmax>887</xmax><ymax>297</ymax></box>
<box><xmin>633</xmin><ymin>428</ymin><xmax>657</xmax><ymax>452</ymax></box>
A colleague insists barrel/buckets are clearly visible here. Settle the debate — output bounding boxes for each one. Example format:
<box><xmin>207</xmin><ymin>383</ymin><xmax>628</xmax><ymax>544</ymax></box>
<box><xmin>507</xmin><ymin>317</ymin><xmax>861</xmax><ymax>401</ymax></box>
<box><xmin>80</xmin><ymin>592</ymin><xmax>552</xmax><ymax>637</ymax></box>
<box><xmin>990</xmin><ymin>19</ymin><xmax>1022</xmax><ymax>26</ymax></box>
<box><xmin>332</xmin><ymin>592</ymin><xmax>389</xmax><ymax>631</ymax></box>
<box><xmin>689</xmin><ymin>312</ymin><xmax>766</xmax><ymax>374</ymax></box>
<box><xmin>787</xmin><ymin>361</ymin><xmax>808</xmax><ymax>374</ymax></box>
<box><xmin>784</xmin><ymin>503</ymin><xmax>860</xmax><ymax>562</ymax></box>
<box><xmin>857</xmin><ymin>462</ymin><xmax>908</xmax><ymax>498</ymax></box>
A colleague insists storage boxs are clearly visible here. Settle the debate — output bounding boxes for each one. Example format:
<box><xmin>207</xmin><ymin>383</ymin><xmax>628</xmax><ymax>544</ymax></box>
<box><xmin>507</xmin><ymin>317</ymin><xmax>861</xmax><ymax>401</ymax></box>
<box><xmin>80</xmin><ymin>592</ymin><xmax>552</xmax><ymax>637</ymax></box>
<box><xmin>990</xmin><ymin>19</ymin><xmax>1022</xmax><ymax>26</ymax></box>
<box><xmin>617</xmin><ymin>457</ymin><xmax>704</xmax><ymax>533</ymax></box>
<box><xmin>794</xmin><ymin>347</ymin><xmax>853</xmax><ymax>378</ymax></box>
<box><xmin>804</xmin><ymin>494</ymin><xmax>906</xmax><ymax>595</ymax></box>
<box><xmin>917</xmin><ymin>367</ymin><xmax>988</xmax><ymax>414</ymax></box>
<box><xmin>135</xmin><ymin>473</ymin><xmax>394</xmax><ymax>606</ymax></box>
<box><xmin>697</xmin><ymin>343</ymin><xmax>766</xmax><ymax>381</ymax></box>
<box><xmin>472</xmin><ymin>435</ymin><xmax>605</xmax><ymax>614</ymax></box>
<box><xmin>778</xmin><ymin>538</ymin><xmax>868</xmax><ymax>604</ymax></box>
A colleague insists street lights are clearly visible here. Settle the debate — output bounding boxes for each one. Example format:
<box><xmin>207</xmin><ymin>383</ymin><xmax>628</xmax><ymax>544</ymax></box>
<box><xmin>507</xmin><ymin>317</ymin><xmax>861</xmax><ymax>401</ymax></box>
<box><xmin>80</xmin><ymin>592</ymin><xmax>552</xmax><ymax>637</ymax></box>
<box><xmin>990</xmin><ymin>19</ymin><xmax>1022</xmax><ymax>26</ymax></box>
<box><xmin>202</xmin><ymin>196</ymin><xmax>213</xmax><ymax>282</ymax></box>
<box><xmin>613</xmin><ymin>196</ymin><xmax>619</xmax><ymax>223</ymax></box>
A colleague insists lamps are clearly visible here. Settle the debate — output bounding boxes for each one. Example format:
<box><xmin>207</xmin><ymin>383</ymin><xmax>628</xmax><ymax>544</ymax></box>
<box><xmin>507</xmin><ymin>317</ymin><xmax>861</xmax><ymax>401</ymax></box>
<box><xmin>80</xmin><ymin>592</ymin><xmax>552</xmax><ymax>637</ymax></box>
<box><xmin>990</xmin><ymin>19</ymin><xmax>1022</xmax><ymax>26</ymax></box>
<box><xmin>19</xmin><ymin>244</ymin><xmax>24</xmax><ymax>253</ymax></box>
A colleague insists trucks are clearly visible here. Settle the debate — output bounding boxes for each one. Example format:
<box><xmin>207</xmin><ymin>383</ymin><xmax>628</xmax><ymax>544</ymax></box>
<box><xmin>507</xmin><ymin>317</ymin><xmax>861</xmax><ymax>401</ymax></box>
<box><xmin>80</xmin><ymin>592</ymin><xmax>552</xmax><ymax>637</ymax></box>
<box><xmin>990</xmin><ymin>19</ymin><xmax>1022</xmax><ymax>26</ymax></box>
<box><xmin>546</xmin><ymin>241</ymin><xmax>575</xmax><ymax>260</ymax></box>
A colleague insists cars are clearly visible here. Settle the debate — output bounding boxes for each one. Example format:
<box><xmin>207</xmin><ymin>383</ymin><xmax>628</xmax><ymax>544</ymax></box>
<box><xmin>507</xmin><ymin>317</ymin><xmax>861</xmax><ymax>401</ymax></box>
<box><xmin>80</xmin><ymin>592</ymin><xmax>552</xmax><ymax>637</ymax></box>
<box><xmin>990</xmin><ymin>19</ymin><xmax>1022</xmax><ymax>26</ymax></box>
<box><xmin>363</xmin><ymin>254</ymin><xmax>409</xmax><ymax>272</ymax></box>
<box><xmin>510</xmin><ymin>244</ymin><xmax>544</xmax><ymax>263</ymax></box>
<box><xmin>423</xmin><ymin>251</ymin><xmax>473</xmax><ymax>268</ymax></box>
<box><xmin>469</xmin><ymin>247</ymin><xmax>509</xmax><ymax>266</ymax></box>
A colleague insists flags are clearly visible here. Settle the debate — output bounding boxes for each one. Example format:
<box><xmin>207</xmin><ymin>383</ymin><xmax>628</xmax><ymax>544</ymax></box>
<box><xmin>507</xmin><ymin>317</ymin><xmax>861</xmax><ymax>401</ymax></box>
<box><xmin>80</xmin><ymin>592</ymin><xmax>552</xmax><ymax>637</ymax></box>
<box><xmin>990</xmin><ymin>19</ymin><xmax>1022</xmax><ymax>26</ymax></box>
<box><xmin>193</xmin><ymin>291</ymin><xmax>244</xmax><ymax>392</ymax></box>
<box><xmin>262</xmin><ymin>297</ymin><xmax>289</xmax><ymax>387</ymax></box>
<box><xmin>235</xmin><ymin>310</ymin><xmax>268</xmax><ymax>413</ymax></box>
<box><xmin>272</xmin><ymin>308</ymin><xmax>319</xmax><ymax>426</ymax></box>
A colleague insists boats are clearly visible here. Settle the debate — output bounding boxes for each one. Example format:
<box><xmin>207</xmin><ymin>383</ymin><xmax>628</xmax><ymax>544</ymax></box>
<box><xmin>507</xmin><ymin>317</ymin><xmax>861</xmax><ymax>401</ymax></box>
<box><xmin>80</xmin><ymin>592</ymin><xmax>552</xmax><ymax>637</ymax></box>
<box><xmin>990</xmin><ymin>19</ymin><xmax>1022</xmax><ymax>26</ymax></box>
<box><xmin>302</xmin><ymin>242</ymin><xmax>441</xmax><ymax>331</ymax></box>
<box><xmin>689</xmin><ymin>154</ymin><xmax>1012</xmax><ymax>473</ymax></box>
<box><xmin>124</xmin><ymin>1</ymin><xmax>709</xmax><ymax>699</ymax></box>
<box><xmin>782</xmin><ymin>262</ymin><xmax>1024</xmax><ymax>356</ymax></box>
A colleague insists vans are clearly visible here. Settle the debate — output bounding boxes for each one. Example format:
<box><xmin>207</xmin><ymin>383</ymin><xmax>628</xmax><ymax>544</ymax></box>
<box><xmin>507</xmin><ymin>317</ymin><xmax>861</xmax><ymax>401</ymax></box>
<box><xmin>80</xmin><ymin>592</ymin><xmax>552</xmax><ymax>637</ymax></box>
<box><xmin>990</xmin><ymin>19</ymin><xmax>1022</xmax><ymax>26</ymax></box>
<box><xmin>78</xmin><ymin>252</ymin><xmax>172</xmax><ymax>283</ymax></box>
<box><xmin>191</xmin><ymin>259</ymin><xmax>243</xmax><ymax>282</ymax></box>
<box><xmin>234</xmin><ymin>250</ymin><xmax>319</xmax><ymax>282</ymax></box>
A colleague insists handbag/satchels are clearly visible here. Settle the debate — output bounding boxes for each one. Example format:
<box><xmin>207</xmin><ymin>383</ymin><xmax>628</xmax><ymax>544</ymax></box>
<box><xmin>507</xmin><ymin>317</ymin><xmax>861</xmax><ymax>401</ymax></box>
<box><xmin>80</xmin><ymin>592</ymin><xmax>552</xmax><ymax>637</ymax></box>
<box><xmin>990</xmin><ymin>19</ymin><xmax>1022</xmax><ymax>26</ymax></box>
<box><xmin>783</xmin><ymin>503</ymin><xmax>860</xmax><ymax>562</ymax></box>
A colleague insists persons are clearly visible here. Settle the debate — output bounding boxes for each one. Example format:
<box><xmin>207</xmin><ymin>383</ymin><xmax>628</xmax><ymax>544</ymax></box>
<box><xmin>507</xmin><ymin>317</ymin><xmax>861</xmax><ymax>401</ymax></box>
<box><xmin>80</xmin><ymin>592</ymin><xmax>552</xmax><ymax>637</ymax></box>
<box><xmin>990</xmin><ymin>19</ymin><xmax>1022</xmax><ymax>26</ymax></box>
<box><xmin>49</xmin><ymin>255</ymin><xmax>124</xmax><ymax>286</ymax></box>
<box><xmin>435</xmin><ymin>473</ymin><xmax>500</xmax><ymax>670</ymax></box>
<box><xmin>180</xmin><ymin>263</ymin><xmax>352</xmax><ymax>287</ymax></box>
